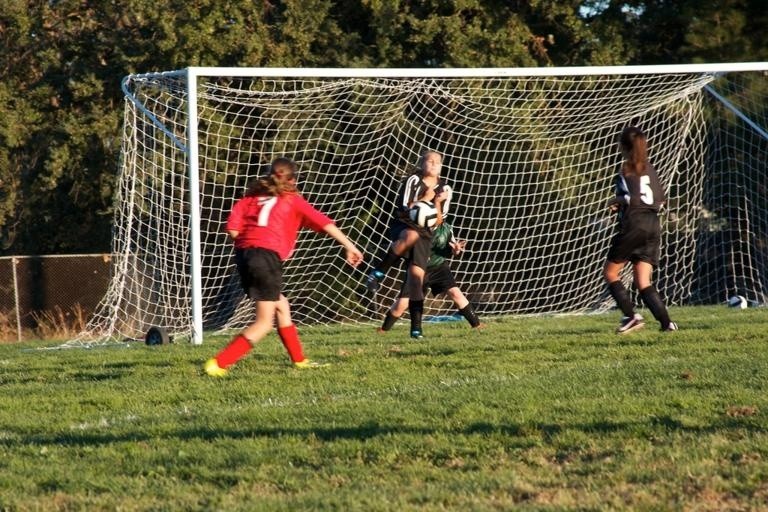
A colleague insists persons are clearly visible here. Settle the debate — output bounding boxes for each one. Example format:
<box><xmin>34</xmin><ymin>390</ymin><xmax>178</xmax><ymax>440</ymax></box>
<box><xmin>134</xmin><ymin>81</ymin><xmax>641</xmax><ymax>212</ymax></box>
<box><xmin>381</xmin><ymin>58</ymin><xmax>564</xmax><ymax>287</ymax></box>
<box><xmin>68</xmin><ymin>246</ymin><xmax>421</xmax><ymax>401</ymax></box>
<box><xmin>604</xmin><ymin>127</ymin><xmax>680</xmax><ymax>335</ymax></box>
<box><xmin>366</xmin><ymin>150</ymin><xmax>453</xmax><ymax>340</ymax></box>
<box><xmin>204</xmin><ymin>158</ymin><xmax>364</xmax><ymax>376</ymax></box>
<box><xmin>376</xmin><ymin>213</ymin><xmax>490</xmax><ymax>333</ymax></box>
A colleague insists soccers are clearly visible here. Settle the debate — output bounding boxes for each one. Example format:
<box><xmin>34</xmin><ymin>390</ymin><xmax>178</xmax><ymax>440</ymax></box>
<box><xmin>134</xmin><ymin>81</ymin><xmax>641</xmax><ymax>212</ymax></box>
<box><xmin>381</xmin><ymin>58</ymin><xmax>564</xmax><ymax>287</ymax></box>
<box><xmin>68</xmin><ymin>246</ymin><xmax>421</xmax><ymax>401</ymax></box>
<box><xmin>410</xmin><ymin>201</ymin><xmax>437</xmax><ymax>228</ymax></box>
<box><xmin>727</xmin><ymin>296</ymin><xmax>748</xmax><ymax>309</ymax></box>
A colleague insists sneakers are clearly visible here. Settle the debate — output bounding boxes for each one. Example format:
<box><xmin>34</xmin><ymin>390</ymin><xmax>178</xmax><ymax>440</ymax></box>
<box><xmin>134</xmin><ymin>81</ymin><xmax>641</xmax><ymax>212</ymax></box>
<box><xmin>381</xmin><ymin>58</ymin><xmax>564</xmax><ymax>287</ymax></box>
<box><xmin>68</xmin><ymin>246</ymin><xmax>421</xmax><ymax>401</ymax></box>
<box><xmin>291</xmin><ymin>358</ymin><xmax>331</xmax><ymax>371</ymax></box>
<box><xmin>411</xmin><ymin>329</ymin><xmax>424</xmax><ymax>339</ymax></box>
<box><xmin>613</xmin><ymin>313</ymin><xmax>646</xmax><ymax>336</ymax></box>
<box><xmin>658</xmin><ymin>321</ymin><xmax>679</xmax><ymax>333</ymax></box>
<box><xmin>366</xmin><ymin>270</ymin><xmax>384</xmax><ymax>291</ymax></box>
<box><xmin>377</xmin><ymin>326</ymin><xmax>387</xmax><ymax>337</ymax></box>
<box><xmin>199</xmin><ymin>358</ymin><xmax>229</xmax><ymax>377</ymax></box>
